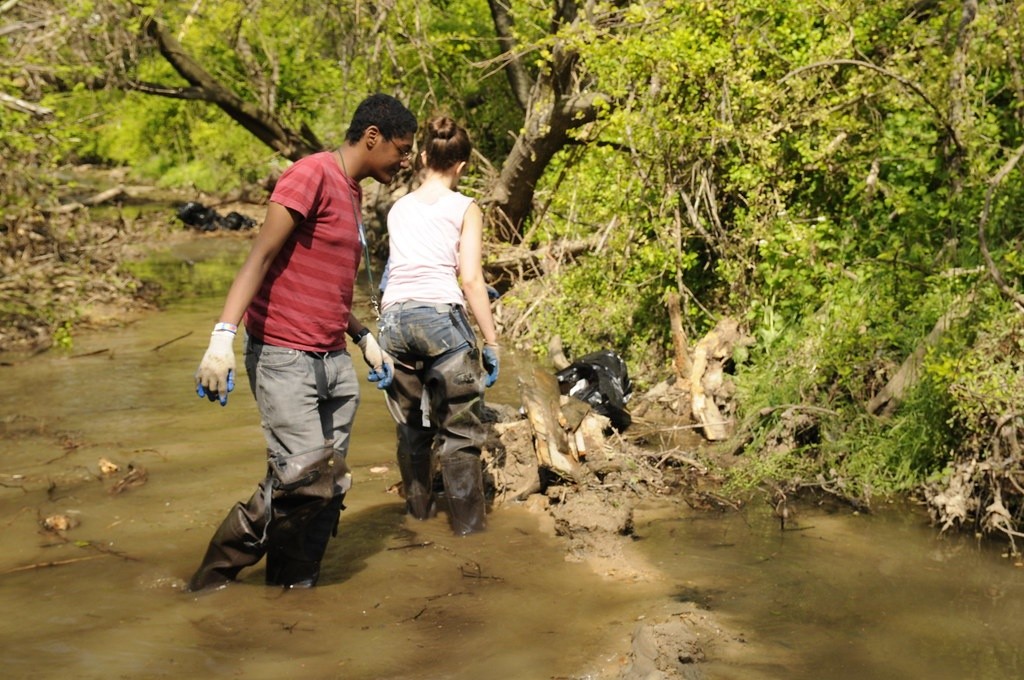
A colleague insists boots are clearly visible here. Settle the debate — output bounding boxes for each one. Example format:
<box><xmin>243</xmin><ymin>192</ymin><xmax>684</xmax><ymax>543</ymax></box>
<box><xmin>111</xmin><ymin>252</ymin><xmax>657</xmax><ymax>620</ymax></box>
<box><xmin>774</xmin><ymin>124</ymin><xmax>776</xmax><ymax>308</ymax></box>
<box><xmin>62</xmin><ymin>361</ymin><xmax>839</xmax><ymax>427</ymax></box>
<box><xmin>189</xmin><ymin>445</ymin><xmax>353</xmax><ymax>591</ymax></box>
<box><xmin>265</xmin><ymin>507</ymin><xmax>339</xmax><ymax>585</ymax></box>
<box><xmin>397</xmin><ymin>450</ymin><xmax>433</xmax><ymax>520</ymax></box>
<box><xmin>439</xmin><ymin>454</ymin><xmax>487</xmax><ymax>535</ymax></box>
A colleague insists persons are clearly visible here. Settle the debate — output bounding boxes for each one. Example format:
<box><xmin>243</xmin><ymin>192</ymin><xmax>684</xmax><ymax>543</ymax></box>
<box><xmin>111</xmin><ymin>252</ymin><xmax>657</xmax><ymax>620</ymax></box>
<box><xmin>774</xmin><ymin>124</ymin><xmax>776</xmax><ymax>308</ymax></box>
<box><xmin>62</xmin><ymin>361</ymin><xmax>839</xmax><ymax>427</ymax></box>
<box><xmin>376</xmin><ymin>115</ymin><xmax>500</xmax><ymax>537</ymax></box>
<box><xmin>185</xmin><ymin>96</ymin><xmax>395</xmax><ymax>596</ymax></box>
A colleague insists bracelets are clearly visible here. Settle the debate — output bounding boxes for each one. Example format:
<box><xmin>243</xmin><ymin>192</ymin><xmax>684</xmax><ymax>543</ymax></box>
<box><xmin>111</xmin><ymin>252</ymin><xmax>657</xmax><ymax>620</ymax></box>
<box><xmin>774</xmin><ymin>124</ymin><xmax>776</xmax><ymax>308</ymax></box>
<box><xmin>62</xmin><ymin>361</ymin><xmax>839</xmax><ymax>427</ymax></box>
<box><xmin>214</xmin><ymin>323</ymin><xmax>238</xmax><ymax>334</ymax></box>
<box><xmin>482</xmin><ymin>339</ymin><xmax>498</xmax><ymax>347</ymax></box>
<box><xmin>352</xmin><ymin>327</ymin><xmax>370</xmax><ymax>345</ymax></box>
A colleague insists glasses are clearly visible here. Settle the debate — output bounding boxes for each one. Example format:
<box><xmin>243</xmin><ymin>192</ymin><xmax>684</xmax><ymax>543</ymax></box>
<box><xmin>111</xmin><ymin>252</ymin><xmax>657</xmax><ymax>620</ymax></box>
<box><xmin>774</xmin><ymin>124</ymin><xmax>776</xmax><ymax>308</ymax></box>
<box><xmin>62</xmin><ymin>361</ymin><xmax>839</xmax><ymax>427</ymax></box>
<box><xmin>383</xmin><ymin>135</ymin><xmax>414</xmax><ymax>160</ymax></box>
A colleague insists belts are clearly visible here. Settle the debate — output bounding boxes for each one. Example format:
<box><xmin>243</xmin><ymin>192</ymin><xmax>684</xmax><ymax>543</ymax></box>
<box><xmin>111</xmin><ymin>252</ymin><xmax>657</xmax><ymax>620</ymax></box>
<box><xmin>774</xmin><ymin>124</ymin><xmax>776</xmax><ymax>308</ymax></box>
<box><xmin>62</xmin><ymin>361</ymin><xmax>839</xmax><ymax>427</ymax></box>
<box><xmin>382</xmin><ymin>301</ymin><xmax>456</xmax><ymax>314</ymax></box>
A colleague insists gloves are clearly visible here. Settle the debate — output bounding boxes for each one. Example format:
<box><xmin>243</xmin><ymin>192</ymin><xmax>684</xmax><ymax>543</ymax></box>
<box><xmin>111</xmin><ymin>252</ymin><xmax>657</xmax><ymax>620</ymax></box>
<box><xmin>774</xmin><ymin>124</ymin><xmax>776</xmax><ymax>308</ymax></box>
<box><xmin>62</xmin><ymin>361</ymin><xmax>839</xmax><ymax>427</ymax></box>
<box><xmin>486</xmin><ymin>285</ymin><xmax>500</xmax><ymax>299</ymax></box>
<box><xmin>482</xmin><ymin>339</ymin><xmax>498</xmax><ymax>387</ymax></box>
<box><xmin>194</xmin><ymin>323</ymin><xmax>239</xmax><ymax>406</ymax></box>
<box><xmin>357</xmin><ymin>333</ymin><xmax>395</xmax><ymax>390</ymax></box>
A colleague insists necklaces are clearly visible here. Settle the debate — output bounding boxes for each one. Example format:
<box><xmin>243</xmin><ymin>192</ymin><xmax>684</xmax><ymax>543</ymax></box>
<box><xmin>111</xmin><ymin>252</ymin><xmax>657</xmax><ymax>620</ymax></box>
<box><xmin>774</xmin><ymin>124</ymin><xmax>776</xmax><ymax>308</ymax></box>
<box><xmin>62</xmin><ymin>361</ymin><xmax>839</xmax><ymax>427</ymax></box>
<box><xmin>338</xmin><ymin>148</ymin><xmax>383</xmax><ymax>322</ymax></box>
